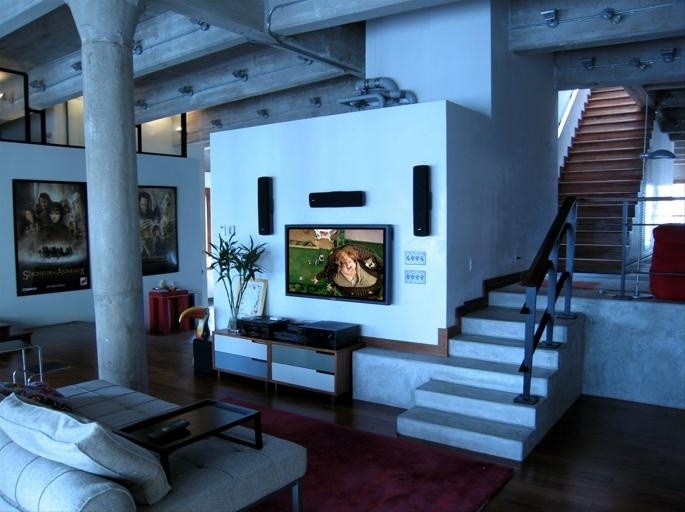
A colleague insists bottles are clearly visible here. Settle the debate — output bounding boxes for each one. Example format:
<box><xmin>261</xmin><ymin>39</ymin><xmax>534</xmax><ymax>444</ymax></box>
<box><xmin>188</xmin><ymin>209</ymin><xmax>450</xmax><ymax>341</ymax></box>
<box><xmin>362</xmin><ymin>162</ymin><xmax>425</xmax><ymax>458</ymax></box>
<box><xmin>169</xmin><ymin>281</ymin><xmax>176</xmax><ymax>296</ymax></box>
<box><xmin>159</xmin><ymin>280</ymin><xmax>164</xmax><ymax>288</ymax></box>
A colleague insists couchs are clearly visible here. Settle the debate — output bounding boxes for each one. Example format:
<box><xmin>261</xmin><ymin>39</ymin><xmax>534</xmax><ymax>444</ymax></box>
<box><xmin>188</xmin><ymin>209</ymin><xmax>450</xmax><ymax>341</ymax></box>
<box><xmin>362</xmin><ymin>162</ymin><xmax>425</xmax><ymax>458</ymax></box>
<box><xmin>0</xmin><ymin>373</ymin><xmax>308</xmax><ymax>512</ymax></box>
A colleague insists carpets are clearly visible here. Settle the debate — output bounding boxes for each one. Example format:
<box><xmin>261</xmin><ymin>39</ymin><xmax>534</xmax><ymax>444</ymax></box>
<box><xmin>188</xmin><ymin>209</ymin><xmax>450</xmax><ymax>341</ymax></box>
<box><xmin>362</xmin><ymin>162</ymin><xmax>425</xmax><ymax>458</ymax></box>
<box><xmin>204</xmin><ymin>396</ymin><xmax>515</xmax><ymax>512</ymax></box>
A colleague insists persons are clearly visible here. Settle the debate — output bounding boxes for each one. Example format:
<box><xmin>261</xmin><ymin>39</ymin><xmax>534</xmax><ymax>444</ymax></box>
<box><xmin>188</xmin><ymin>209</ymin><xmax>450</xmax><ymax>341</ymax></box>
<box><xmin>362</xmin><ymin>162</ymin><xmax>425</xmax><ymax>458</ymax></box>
<box><xmin>151</xmin><ymin>224</ymin><xmax>168</xmax><ymax>257</ymax></box>
<box><xmin>138</xmin><ymin>192</ymin><xmax>158</xmax><ymax>257</ymax></box>
<box><xmin>18</xmin><ymin>191</ymin><xmax>76</xmax><ymax>258</ymax></box>
<box><xmin>159</xmin><ymin>192</ymin><xmax>175</xmax><ymax>249</ymax></box>
<box><xmin>311</xmin><ymin>245</ymin><xmax>383</xmax><ymax>299</ymax></box>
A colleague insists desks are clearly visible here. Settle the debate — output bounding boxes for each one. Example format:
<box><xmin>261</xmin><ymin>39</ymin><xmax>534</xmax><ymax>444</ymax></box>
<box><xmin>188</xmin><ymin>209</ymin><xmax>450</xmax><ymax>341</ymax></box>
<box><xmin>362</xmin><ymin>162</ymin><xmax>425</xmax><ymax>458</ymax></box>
<box><xmin>0</xmin><ymin>337</ymin><xmax>45</xmax><ymax>390</ymax></box>
<box><xmin>111</xmin><ymin>395</ymin><xmax>264</xmax><ymax>483</ymax></box>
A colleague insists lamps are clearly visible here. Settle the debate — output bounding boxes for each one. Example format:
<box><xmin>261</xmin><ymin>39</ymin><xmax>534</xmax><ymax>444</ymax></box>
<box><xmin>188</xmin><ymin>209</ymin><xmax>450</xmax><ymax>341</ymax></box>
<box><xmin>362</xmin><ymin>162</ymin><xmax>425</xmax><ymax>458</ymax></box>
<box><xmin>29</xmin><ymin>17</ymin><xmax>321</xmax><ymax>125</ymax></box>
<box><xmin>628</xmin><ymin>149</ymin><xmax>675</xmax><ymax>300</ymax></box>
<box><xmin>540</xmin><ymin>7</ymin><xmax>675</xmax><ymax>71</ymax></box>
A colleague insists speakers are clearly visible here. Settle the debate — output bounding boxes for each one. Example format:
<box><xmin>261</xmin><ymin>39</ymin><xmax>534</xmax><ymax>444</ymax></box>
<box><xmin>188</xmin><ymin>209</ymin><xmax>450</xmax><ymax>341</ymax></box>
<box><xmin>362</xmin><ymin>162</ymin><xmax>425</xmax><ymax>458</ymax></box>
<box><xmin>257</xmin><ymin>177</ymin><xmax>273</xmax><ymax>235</ymax></box>
<box><xmin>412</xmin><ymin>164</ymin><xmax>431</xmax><ymax>237</ymax></box>
<box><xmin>309</xmin><ymin>191</ymin><xmax>364</xmax><ymax>207</ymax></box>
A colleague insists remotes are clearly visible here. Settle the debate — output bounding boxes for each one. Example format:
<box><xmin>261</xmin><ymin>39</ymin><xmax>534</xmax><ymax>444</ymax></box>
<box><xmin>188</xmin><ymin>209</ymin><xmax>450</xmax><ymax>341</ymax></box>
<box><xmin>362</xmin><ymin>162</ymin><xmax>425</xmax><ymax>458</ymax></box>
<box><xmin>146</xmin><ymin>418</ymin><xmax>191</xmax><ymax>439</ymax></box>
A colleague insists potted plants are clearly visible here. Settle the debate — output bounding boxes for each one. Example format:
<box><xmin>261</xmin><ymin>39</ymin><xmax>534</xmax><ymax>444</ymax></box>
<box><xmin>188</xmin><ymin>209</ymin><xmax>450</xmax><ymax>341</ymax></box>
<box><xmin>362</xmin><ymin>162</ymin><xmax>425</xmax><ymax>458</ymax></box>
<box><xmin>203</xmin><ymin>227</ymin><xmax>270</xmax><ymax>333</ymax></box>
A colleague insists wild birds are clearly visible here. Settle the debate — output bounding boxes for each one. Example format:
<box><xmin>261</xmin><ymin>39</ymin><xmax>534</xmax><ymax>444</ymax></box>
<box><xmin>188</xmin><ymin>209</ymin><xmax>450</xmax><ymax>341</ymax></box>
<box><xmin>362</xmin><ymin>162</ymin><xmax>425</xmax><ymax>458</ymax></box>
<box><xmin>177</xmin><ymin>306</ymin><xmax>211</xmax><ymax>340</ymax></box>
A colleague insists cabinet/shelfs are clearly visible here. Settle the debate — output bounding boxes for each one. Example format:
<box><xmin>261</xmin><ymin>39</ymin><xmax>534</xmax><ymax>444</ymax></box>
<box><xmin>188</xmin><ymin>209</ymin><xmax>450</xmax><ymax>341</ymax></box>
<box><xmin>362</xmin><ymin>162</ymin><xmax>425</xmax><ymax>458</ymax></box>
<box><xmin>210</xmin><ymin>325</ymin><xmax>363</xmax><ymax>401</ymax></box>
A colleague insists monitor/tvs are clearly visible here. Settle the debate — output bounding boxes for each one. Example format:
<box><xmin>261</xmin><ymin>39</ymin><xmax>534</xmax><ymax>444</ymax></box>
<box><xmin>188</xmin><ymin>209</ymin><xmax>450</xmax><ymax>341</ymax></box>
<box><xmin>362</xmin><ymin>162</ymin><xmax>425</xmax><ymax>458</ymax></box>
<box><xmin>284</xmin><ymin>224</ymin><xmax>392</xmax><ymax>306</ymax></box>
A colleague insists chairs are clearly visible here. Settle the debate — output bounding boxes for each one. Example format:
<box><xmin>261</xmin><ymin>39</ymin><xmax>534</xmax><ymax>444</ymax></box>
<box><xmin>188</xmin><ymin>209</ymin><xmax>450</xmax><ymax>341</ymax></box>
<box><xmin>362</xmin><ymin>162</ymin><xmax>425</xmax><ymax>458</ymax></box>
<box><xmin>646</xmin><ymin>223</ymin><xmax>685</xmax><ymax>300</ymax></box>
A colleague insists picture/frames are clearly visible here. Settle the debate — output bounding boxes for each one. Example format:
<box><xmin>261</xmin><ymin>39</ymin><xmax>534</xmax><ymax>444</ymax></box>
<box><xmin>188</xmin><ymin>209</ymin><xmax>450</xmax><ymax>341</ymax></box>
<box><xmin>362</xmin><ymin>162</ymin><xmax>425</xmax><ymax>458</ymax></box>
<box><xmin>239</xmin><ymin>277</ymin><xmax>267</xmax><ymax>319</ymax></box>
<box><xmin>13</xmin><ymin>179</ymin><xmax>179</xmax><ymax>296</ymax></box>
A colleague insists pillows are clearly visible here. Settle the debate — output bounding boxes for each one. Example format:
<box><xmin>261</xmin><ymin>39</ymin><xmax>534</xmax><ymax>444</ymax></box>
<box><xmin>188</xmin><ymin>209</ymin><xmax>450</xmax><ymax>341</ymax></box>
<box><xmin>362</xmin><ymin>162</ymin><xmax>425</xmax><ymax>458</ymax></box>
<box><xmin>3</xmin><ymin>379</ymin><xmax>72</xmax><ymax>413</ymax></box>
